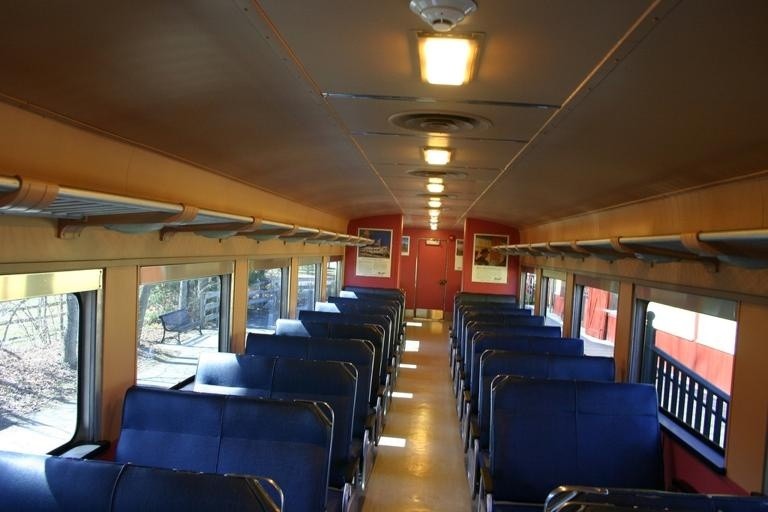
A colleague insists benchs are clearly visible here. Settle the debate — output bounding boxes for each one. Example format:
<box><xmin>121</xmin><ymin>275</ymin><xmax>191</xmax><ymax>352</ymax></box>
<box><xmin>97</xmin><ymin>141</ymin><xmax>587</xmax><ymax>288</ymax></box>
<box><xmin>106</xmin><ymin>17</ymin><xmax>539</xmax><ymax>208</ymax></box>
<box><xmin>192</xmin><ymin>284</ymin><xmax>408</xmax><ymax>490</ymax></box>
<box><xmin>449</xmin><ymin>293</ymin><xmax>616</xmax><ymax>501</ymax></box>
<box><xmin>545</xmin><ymin>485</ymin><xmax>767</xmax><ymax>511</ymax></box>
<box><xmin>477</xmin><ymin>375</ymin><xmax>662</xmax><ymax>511</ymax></box>
<box><xmin>1</xmin><ymin>448</ymin><xmax>284</xmax><ymax>511</ymax></box>
<box><xmin>115</xmin><ymin>384</ymin><xmax>351</xmax><ymax>511</ymax></box>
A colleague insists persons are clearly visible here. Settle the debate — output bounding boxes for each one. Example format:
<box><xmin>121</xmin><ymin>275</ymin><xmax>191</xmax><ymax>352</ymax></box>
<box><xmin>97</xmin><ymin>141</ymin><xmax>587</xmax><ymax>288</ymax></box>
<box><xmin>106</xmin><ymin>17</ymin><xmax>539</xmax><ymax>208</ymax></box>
<box><xmin>474</xmin><ymin>247</ymin><xmax>490</xmax><ymax>266</ymax></box>
